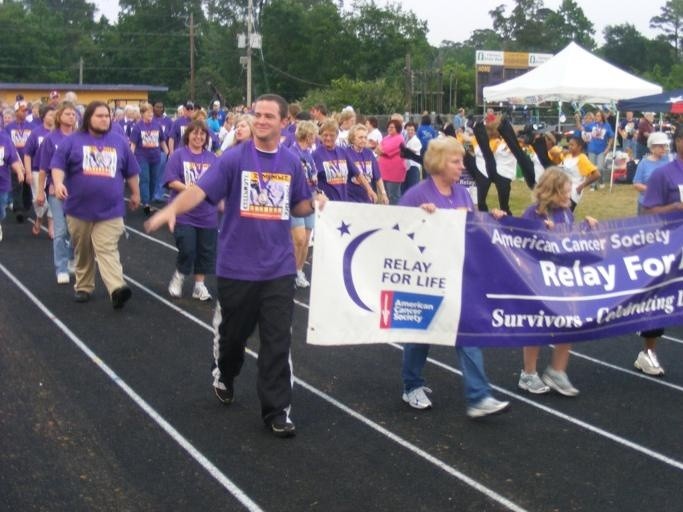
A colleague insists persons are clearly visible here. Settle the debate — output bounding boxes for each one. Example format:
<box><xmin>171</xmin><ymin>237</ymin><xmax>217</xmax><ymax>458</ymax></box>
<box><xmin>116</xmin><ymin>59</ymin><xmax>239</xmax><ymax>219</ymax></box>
<box><xmin>0</xmin><ymin>91</ymin><xmax>683</xmax><ymax>439</ymax></box>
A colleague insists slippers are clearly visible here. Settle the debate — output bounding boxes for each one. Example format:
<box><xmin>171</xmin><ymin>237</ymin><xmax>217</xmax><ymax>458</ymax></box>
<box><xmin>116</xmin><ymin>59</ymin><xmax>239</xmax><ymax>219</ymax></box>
<box><xmin>32</xmin><ymin>220</ymin><xmax>43</xmax><ymax>235</ymax></box>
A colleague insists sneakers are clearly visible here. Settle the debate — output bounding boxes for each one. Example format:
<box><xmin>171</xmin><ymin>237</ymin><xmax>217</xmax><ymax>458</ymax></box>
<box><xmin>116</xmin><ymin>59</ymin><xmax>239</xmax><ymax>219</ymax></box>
<box><xmin>465</xmin><ymin>392</ymin><xmax>511</xmax><ymax>418</ymax></box>
<box><xmin>56</xmin><ymin>260</ymin><xmax>76</xmax><ymax>284</ymax></box>
<box><xmin>112</xmin><ymin>286</ymin><xmax>132</xmax><ymax>310</ymax></box>
<box><xmin>74</xmin><ymin>291</ymin><xmax>88</xmax><ymax>302</ymax></box>
<box><xmin>635</xmin><ymin>347</ymin><xmax>664</xmax><ymax>377</ymax></box>
<box><xmin>168</xmin><ymin>269</ymin><xmax>185</xmax><ymax>298</ymax></box>
<box><xmin>403</xmin><ymin>387</ymin><xmax>431</xmax><ymax>413</ymax></box>
<box><xmin>209</xmin><ymin>362</ymin><xmax>235</xmax><ymax>403</ymax></box>
<box><xmin>519</xmin><ymin>369</ymin><xmax>581</xmax><ymax>400</ymax></box>
<box><xmin>296</xmin><ymin>269</ymin><xmax>310</xmax><ymax>288</ymax></box>
<box><xmin>271</xmin><ymin>417</ymin><xmax>297</xmax><ymax>438</ymax></box>
<box><xmin>192</xmin><ymin>283</ymin><xmax>213</xmax><ymax>302</ymax></box>
